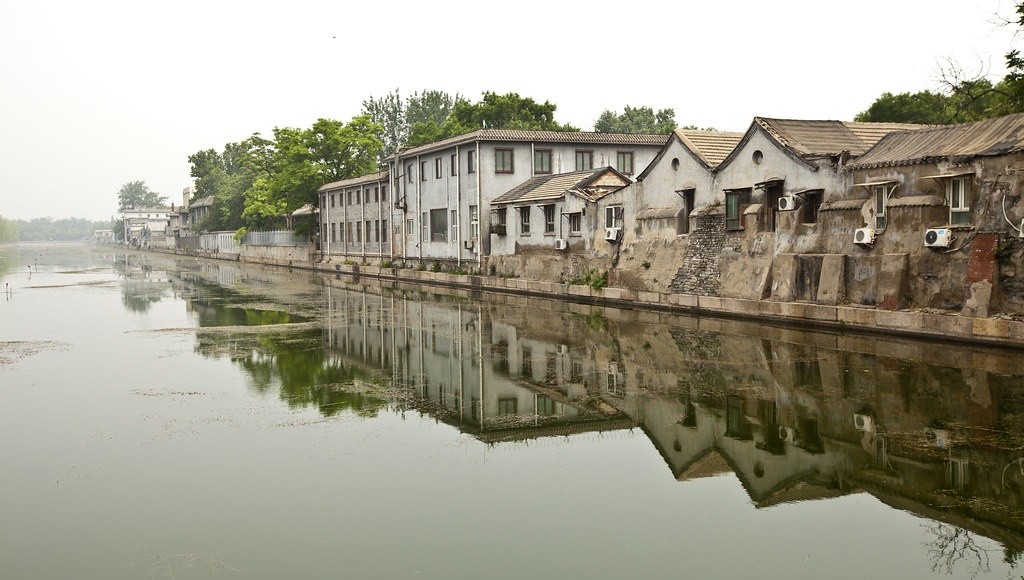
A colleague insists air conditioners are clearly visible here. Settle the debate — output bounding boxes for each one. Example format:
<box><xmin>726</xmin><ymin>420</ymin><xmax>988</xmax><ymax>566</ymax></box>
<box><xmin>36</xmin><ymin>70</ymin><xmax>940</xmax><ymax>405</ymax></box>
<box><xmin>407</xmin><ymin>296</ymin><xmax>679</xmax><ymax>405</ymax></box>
<box><xmin>554</xmin><ymin>238</ymin><xmax>567</xmax><ymax>249</ymax></box>
<box><xmin>605</xmin><ymin>228</ymin><xmax>618</xmax><ymax>240</ymax></box>
<box><xmin>607</xmin><ymin>363</ymin><xmax>618</xmax><ymax>375</ymax></box>
<box><xmin>854</xmin><ymin>412</ymin><xmax>875</xmax><ymax>431</ymax></box>
<box><xmin>853</xmin><ymin>228</ymin><xmax>875</xmax><ymax>244</ymax></box>
<box><xmin>463</xmin><ymin>240</ymin><xmax>473</xmax><ymax>248</ymax></box>
<box><xmin>922</xmin><ymin>428</ymin><xmax>950</xmax><ymax>450</ymax></box>
<box><xmin>778</xmin><ymin>196</ymin><xmax>796</xmax><ymax>211</ymax></box>
<box><xmin>924</xmin><ymin>229</ymin><xmax>952</xmax><ymax>247</ymax></box>
<box><xmin>556</xmin><ymin>343</ymin><xmax>568</xmax><ymax>354</ymax></box>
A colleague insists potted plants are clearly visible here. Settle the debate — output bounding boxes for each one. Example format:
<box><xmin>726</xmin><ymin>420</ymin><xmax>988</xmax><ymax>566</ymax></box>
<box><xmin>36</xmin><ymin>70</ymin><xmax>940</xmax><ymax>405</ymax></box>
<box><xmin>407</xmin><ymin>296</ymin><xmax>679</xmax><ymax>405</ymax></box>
<box><xmin>489</xmin><ymin>222</ymin><xmax>506</xmax><ymax>236</ymax></box>
